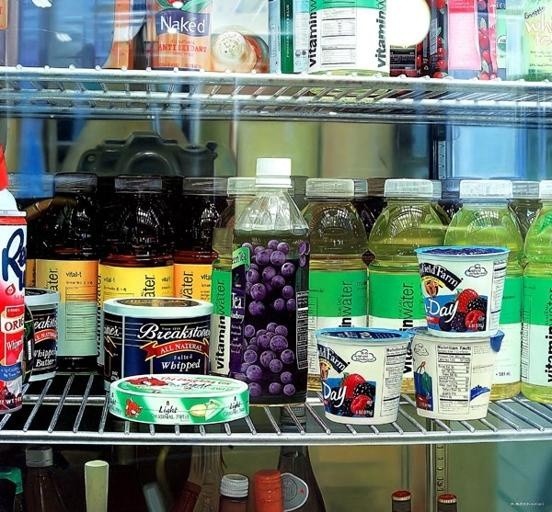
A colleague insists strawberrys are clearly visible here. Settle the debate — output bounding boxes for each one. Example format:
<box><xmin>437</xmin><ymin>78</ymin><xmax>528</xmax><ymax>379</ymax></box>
<box><xmin>454</xmin><ymin>288</ymin><xmax>478</xmax><ymax>312</ymax></box>
<box><xmin>350</xmin><ymin>395</ymin><xmax>372</xmax><ymax>415</ymax></box>
<box><xmin>465</xmin><ymin>309</ymin><xmax>485</xmax><ymax>331</ymax></box>
<box><xmin>341</xmin><ymin>372</ymin><xmax>365</xmax><ymax>399</ymax></box>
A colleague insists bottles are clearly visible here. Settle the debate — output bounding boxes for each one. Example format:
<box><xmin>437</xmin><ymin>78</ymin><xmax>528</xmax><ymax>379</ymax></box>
<box><xmin>102</xmin><ymin>294</ymin><xmax>213</xmax><ymax>392</ymax></box>
<box><xmin>230</xmin><ymin>158</ymin><xmax>311</xmax><ymax>405</ymax></box>
<box><xmin>34</xmin><ymin>173</ymin><xmax>101</xmax><ymax>375</ymax></box>
<box><xmin>0</xmin><ymin>443</ymin><xmax>330</xmax><ymax>512</ymax></box>
<box><xmin>289</xmin><ymin>172</ymin><xmax>552</xmax><ymax>407</ymax></box>
<box><xmin>211</xmin><ymin>175</ymin><xmax>258</xmax><ymax>375</ymax></box>
<box><xmin>10</xmin><ymin>169</ymin><xmax>39</xmax><ymax>287</ymax></box>
<box><xmin>215</xmin><ymin>0</ymin><xmax>551</xmax><ymax>82</ymax></box>
<box><xmin>99</xmin><ymin>172</ymin><xmax>175</xmax><ymax>370</ymax></box>
<box><xmin>0</xmin><ymin>144</ymin><xmax>29</xmax><ymax>417</ymax></box>
<box><xmin>22</xmin><ymin>287</ymin><xmax>62</xmax><ymax>383</ymax></box>
<box><xmin>174</xmin><ymin>175</ymin><xmax>227</xmax><ymax>306</ymax></box>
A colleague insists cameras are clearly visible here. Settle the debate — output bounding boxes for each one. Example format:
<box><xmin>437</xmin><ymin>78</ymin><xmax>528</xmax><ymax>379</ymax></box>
<box><xmin>75</xmin><ymin>132</ymin><xmax>219</xmax><ymax>177</ymax></box>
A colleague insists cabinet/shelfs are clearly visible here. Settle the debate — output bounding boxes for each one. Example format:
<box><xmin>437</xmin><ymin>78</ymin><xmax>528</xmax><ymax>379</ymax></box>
<box><xmin>0</xmin><ymin>61</ymin><xmax>552</xmax><ymax>511</ymax></box>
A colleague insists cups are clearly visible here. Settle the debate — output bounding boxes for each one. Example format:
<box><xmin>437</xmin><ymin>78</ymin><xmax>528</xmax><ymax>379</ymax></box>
<box><xmin>409</xmin><ymin>325</ymin><xmax>504</xmax><ymax>420</ymax></box>
<box><xmin>313</xmin><ymin>327</ymin><xmax>413</xmax><ymax>425</ymax></box>
<box><xmin>414</xmin><ymin>244</ymin><xmax>509</xmax><ymax>340</ymax></box>
